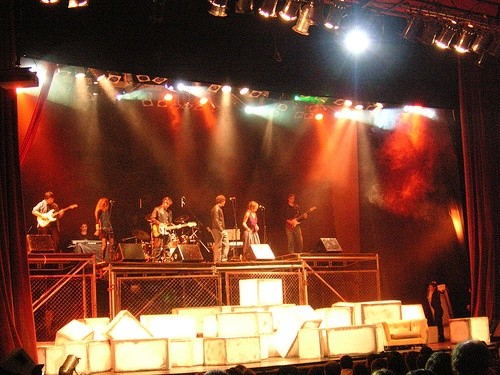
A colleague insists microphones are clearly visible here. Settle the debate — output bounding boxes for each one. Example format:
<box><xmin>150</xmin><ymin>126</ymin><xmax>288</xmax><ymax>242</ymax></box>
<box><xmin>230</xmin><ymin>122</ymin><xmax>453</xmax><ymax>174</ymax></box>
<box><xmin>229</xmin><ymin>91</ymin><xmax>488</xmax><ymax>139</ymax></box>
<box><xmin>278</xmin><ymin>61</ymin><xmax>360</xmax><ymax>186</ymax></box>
<box><xmin>180</xmin><ymin>197</ymin><xmax>184</xmax><ymax>208</ymax></box>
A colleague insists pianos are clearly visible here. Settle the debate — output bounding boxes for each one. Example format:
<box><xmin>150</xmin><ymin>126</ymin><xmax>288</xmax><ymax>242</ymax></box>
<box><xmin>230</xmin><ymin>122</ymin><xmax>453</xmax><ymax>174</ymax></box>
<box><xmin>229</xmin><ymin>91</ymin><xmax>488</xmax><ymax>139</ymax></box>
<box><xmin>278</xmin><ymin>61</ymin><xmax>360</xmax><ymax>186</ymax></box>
<box><xmin>69</xmin><ymin>238</ymin><xmax>109</xmax><ymax>260</ymax></box>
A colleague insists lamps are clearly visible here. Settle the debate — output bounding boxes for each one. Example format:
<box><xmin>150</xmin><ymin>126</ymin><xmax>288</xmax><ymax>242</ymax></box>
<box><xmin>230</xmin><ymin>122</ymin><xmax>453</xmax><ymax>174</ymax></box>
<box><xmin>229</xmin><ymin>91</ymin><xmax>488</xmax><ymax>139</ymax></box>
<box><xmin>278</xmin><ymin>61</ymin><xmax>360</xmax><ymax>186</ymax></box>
<box><xmin>208</xmin><ymin>0</ymin><xmax>228</xmax><ymax>17</ymax></box>
<box><xmin>468</xmin><ymin>28</ymin><xmax>493</xmax><ymax>55</ymax></box>
<box><xmin>234</xmin><ymin>0</ymin><xmax>254</xmax><ymax>12</ymax></box>
<box><xmin>278</xmin><ymin>0</ymin><xmax>301</xmax><ymax>21</ymax></box>
<box><xmin>485</xmin><ymin>33</ymin><xmax>500</xmax><ymax>58</ymax></box>
<box><xmin>418</xmin><ymin>16</ymin><xmax>442</xmax><ymax>46</ymax></box>
<box><xmin>38</xmin><ymin>0</ymin><xmax>59</xmax><ymax>7</ymax></box>
<box><xmin>68</xmin><ymin>0</ymin><xmax>89</xmax><ymax>9</ymax></box>
<box><xmin>435</xmin><ymin>19</ymin><xmax>458</xmax><ymax>48</ymax></box>
<box><xmin>55</xmin><ymin>64</ymin><xmax>401</xmax><ymax>132</ymax></box>
<box><xmin>309</xmin><ymin>0</ymin><xmax>324</xmax><ymax>25</ymax></box>
<box><xmin>291</xmin><ymin>0</ymin><xmax>314</xmax><ymax>36</ymax></box>
<box><xmin>399</xmin><ymin>14</ymin><xmax>423</xmax><ymax>43</ymax></box>
<box><xmin>325</xmin><ymin>3</ymin><xmax>344</xmax><ymax>28</ymax></box>
<box><xmin>258</xmin><ymin>0</ymin><xmax>278</xmax><ymax>16</ymax></box>
<box><xmin>454</xmin><ymin>25</ymin><xmax>476</xmax><ymax>51</ymax></box>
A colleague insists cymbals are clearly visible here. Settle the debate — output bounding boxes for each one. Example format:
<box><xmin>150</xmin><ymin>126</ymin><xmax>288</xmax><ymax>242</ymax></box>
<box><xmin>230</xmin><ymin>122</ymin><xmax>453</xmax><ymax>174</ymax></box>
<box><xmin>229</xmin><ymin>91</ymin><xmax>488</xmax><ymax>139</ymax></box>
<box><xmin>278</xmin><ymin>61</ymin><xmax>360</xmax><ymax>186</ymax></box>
<box><xmin>145</xmin><ymin>214</ymin><xmax>152</xmax><ymax>221</ymax></box>
<box><xmin>174</xmin><ymin>215</ymin><xmax>190</xmax><ymax>224</ymax></box>
<box><xmin>132</xmin><ymin>229</ymin><xmax>150</xmax><ymax>241</ymax></box>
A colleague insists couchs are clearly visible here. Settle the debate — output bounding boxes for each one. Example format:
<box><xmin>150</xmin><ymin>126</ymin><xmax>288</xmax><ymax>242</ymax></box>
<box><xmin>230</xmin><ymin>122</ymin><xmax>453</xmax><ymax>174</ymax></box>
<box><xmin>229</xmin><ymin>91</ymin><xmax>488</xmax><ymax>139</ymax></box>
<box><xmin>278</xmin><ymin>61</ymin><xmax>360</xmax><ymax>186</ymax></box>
<box><xmin>377</xmin><ymin>319</ymin><xmax>429</xmax><ymax>350</ymax></box>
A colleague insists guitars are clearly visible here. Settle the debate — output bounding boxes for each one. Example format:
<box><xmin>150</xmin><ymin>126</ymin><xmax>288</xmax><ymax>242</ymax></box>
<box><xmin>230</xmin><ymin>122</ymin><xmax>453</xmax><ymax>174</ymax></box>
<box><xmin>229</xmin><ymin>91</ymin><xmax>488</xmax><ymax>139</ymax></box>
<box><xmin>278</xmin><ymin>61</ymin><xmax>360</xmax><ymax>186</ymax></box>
<box><xmin>36</xmin><ymin>204</ymin><xmax>78</xmax><ymax>227</ymax></box>
<box><xmin>152</xmin><ymin>221</ymin><xmax>197</xmax><ymax>237</ymax></box>
<box><xmin>285</xmin><ymin>206</ymin><xmax>317</xmax><ymax>230</ymax></box>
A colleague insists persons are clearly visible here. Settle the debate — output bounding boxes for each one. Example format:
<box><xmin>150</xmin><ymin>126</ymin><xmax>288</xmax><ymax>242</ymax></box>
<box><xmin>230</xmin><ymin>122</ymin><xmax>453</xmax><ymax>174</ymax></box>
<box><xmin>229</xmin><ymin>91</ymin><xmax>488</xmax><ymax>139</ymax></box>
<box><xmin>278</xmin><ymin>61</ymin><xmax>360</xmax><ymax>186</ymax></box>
<box><xmin>197</xmin><ymin>339</ymin><xmax>500</xmax><ymax>375</ymax></box>
<box><xmin>76</xmin><ymin>224</ymin><xmax>93</xmax><ymax>240</ymax></box>
<box><xmin>150</xmin><ymin>196</ymin><xmax>176</xmax><ymax>261</ymax></box>
<box><xmin>455</xmin><ymin>288</ymin><xmax>472</xmax><ymax>318</ymax></box>
<box><xmin>95</xmin><ymin>197</ymin><xmax>117</xmax><ymax>262</ymax></box>
<box><xmin>281</xmin><ymin>193</ymin><xmax>308</xmax><ymax>258</ymax></box>
<box><xmin>32</xmin><ymin>191</ymin><xmax>61</xmax><ymax>253</ymax></box>
<box><xmin>241</xmin><ymin>200</ymin><xmax>261</xmax><ymax>262</ymax></box>
<box><xmin>211</xmin><ymin>194</ymin><xmax>229</xmax><ymax>262</ymax></box>
<box><xmin>429</xmin><ymin>282</ymin><xmax>447</xmax><ymax>342</ymax></box>
<box><xmin>0</xmin><ymin>348</ymin><xmax>44</xmax><ymax>375</ymax></box>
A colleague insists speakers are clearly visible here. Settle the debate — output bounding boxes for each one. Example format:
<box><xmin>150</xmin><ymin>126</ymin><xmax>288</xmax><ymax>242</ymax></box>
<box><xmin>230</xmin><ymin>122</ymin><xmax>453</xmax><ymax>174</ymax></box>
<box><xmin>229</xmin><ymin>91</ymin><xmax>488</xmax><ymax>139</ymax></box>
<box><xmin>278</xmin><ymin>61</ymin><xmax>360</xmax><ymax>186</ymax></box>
<box><xmin>26</xmin><ymin>234</ymin><xmax>54</xmax><ymax>254</ymax></box>
<box><xmin>114</xmin><ymin>242</ymin><xmax>147</xmax><ymax>262</ymax></box>
<box><xmin>243</xmin><ymin>243</ymin><xmax>277</xmax><ymax>262</ymax></box>
<box><xmin>314</xmin><ymin>237</ymin><xmax>342</xmax><ymax>254</ymax></box>
<box><xmin>170</xmin><ymin>244</ymin><xmax>204</xmax><ymax>262</ymax></box>
